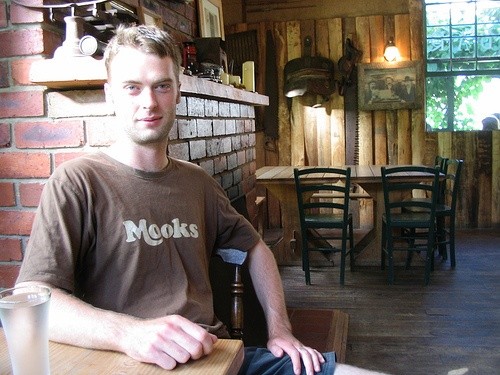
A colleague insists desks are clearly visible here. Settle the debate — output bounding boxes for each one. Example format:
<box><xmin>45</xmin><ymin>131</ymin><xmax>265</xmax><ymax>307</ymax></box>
<box><xmin>255</xmin><ymin>165</ymin><xmax>445</xmax><ymax>266</ymax></box>
<box><xmin>0</xmin><ymin>317</ymin><xmax>245</xmax><ymax>375</ymax></box>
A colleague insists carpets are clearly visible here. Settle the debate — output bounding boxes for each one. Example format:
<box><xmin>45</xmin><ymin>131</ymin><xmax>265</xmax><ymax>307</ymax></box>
<box><xmin>244</xmin><ymin>308</ymin><xmax>349</xmax><ymax>363</ymax></box>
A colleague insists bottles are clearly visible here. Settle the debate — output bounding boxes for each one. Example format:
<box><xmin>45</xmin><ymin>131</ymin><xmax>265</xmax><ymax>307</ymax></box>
<box><xmin>184</xmin><ymin>40</ymin><xmax>196</xmax><ymax>69</ymax></box>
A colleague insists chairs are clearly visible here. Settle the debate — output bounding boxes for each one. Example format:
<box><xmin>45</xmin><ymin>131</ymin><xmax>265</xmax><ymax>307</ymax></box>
<box><xmin>293</xmin><ymin>168</ymin><xmax>355</xmax><ymax>286</ymax></box>
<box><xmin>381</xmin><ymin>165</ymin><xmax>440</xmax><ymax>285</ymax></box>
<box><xmin>404</xmin><ymin>156</ymin><xmax>464</xmax><ymax>269</ymax></box>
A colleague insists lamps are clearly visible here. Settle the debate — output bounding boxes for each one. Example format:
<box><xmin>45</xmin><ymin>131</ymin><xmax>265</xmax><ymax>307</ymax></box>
<box><xmin>383</xmin><ymin>37</ymin><xmax>401</xmax><ymax>62</ymax></box>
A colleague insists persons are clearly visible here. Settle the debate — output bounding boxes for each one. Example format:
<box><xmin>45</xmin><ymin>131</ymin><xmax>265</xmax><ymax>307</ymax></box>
<box><xmin>359</xmin><ymin>63</ymin><xmax>427</xmax><ymax>112</ymax></box>
<box><xmin>12</xmin><ymin>22</ymin><xmax>392</xmax><ymax>375</ymax></box>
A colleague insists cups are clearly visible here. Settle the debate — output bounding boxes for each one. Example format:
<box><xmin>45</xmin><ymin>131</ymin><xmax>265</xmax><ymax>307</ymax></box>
<box><xmin>0</xmin><ymin>285</ymin><xmax>53</xmax><ymax>375</ymax></box>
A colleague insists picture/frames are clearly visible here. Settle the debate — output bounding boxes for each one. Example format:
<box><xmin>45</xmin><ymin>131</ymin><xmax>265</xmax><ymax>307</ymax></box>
<box><xmin>357</xmin><ymin>59</ymin><xmax>424</xmax><ymax>111</ymax></box>
<box><xmin>197</xmin><ymin>0</ymin><xmax>225</xmax><ymax>42</ymax></box>
